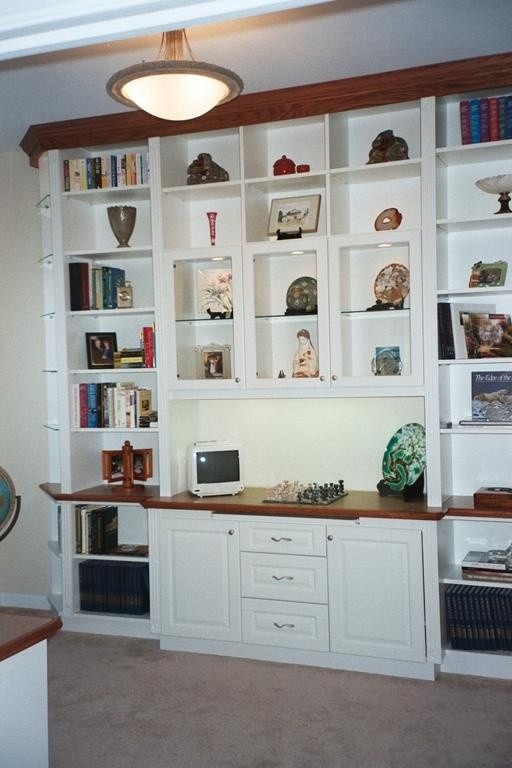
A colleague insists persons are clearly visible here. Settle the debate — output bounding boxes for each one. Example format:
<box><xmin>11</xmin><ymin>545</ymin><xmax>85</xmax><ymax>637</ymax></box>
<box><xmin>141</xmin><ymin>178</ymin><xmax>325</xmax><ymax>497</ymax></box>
<box><xmin>93</xmin><ymin>337</ymin><xmax>113</xmax><ymax>360</ymax></box>
<box><xmin>291</xmin><ymin>329</ymin><xmax>320</xmax><ymax>378</ymax></box>
<box><xmin>209</xmin><ymin>355</ymin><xmax>220</xmax><ymax>373</ymax></box>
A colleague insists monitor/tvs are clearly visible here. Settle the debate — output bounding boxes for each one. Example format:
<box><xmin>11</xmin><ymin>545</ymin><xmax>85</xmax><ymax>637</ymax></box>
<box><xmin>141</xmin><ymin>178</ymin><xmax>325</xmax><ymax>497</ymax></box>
<box><xmin>187</xmin><ymin>443</ymin><xmax>242</xmax><ymax>498</ymax></box>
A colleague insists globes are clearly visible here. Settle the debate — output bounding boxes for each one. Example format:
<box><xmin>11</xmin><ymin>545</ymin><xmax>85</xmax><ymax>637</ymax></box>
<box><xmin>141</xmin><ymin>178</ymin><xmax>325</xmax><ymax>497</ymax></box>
<box><xmin>0</xmin><ymin>465</ymin><xmax>21</xmax><ymax>541</ymax></box>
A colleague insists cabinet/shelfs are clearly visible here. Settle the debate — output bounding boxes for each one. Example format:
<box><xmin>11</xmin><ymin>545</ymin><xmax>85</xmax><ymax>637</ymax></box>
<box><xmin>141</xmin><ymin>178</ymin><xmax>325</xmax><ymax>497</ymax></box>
<box><xmin>17</xmin><ymin>53</ymin><xmax>512</xmax><ymax>685</ymax></box>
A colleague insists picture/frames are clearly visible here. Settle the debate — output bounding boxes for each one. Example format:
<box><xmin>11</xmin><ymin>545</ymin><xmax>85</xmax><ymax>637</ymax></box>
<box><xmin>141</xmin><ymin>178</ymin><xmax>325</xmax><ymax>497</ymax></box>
<box><xmin>459</xmin><ymin>312</ymin><xmax>512</xmax><ymax>359</ymax></box>
<box><xmin>468</xmin><ymin>260</ymin><xmax>507</xmax><ymax>289</ymax></box>
<box><xmin>265</xmin><ymin>194</ymin><xmax>322</xmax><ymax>236</ymax></box>
<box><xmin>192</xmin><ymin>343</ymin><xmax>231</xmax><ymax>380</ymax></box>
<box><xmin>101</xmin><ymin>450</ymin><xmax>154</xmax><ymax>485</ymax></box>
<box><xmin>84</xmin><ymin>331</ymin><xmax>119</xmax><ymax>369</ymax></box>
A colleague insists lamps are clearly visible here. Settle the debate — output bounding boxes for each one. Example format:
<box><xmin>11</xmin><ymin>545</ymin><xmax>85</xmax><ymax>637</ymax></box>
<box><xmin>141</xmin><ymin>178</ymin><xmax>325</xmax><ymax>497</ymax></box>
<box><xmin>101</xmin><ymin>28</ymin><xmax>244</xmax><ymax>125</ymax></box>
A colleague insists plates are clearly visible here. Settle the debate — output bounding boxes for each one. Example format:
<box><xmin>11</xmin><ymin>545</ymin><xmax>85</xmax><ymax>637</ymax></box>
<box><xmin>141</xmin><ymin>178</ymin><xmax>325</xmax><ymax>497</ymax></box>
<box><xmin>383</xmin><ymin>423</ymin><xmax>425</xmax><ymax>490</ymax></box>
<box><xmin>287</xmin><ymin>276</ymin><xmax>317</xmax><ymax>312</ymax></box>
<box><xmin>374</xmin><ymin>264</ymin><xmax>410</xmax><ymax>307</ymax></box>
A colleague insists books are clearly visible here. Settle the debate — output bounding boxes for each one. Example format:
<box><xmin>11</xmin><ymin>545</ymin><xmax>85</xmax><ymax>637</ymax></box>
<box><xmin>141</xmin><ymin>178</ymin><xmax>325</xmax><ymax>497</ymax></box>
<box><xmin>461</xmin><ymin>550</ymin><xmax>508</xmax><ymax>570</ymax></box>
<box><xmin>458</xmin><ymin>419</ymin><xmax>512</xmax><ymax>427</ymax></box>
<box><xmin>57</xmin><ymin>504</ymin><xmax>119</xmax><ymax>554</ymax></box>
<box><xmin>78</xmin><ymin>558</ymin><xmax>150</xmax><ymax>614</ymax></box>
<box><xmin>461</xmin><ymin>572</ymin><xmax>512</xmax><ymax>582</ymax></box>
<box><xmin>458</xmin><ymin>94</ymin><xmax>511</xmax><ymax>145</ymax></box>
<box><xmin>62</xmin><ymin>149</ymin><xmax>151</xmax><ymax>191</ymax></box>
<box><xmin>469</xmin><ymin>370</ymin><xmax>512</xmax><ymax>422</ymax></box>
<box><xmin>68</xmin><ymin>261</ymin><xmax>127</xmax><ymax>310</ymax></box>
<box><xmin>71</xmin><ymin>380</ymin><xmax>158</xmax><ymax>429</ymax></box>
<box><xmin>462</xmin><ymin>567</ymin><xmax>511</xmax><ymax>578</ymax></box>
<box><xmin>444</xmin><ymin>583</ymin><xmax>511</xmax><ymax>654</ymax></box>
<box><xmin>113</xmin><ymin>323</ymin><xmax>157</xmax><ymax>368</ymax></box>
<box><xmin>435</xmin><ymin>300</ymin><xmax>512</xmax><ymax>360</ymax></box>
